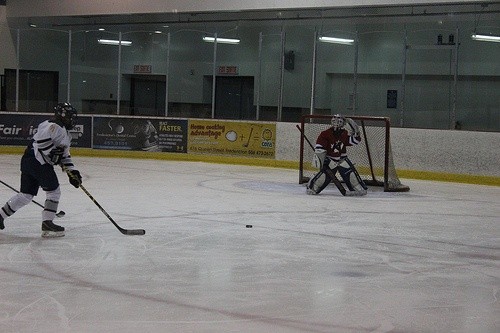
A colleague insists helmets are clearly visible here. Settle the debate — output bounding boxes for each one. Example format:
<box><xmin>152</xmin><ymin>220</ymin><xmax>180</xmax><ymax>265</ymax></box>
<box><xmin>54</xmin><ymin>102</ymin><xmax>78</xmax><ymax>129</ymax></box>
<box><xmin>331</xmin><ymin>114</ymin><xmax>346</xmax><ymax>130</ymax></box>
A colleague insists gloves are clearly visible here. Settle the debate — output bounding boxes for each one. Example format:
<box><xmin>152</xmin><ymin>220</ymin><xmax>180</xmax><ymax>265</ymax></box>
<box><xmin>51</xmin><ymin>147</ymin><xmax>63</xmax><ymax>164</ymax></box>
<box><xmin>68</xmin><ymin>170</ymin><xmax>82</xmax><ymax>188</ymax></box>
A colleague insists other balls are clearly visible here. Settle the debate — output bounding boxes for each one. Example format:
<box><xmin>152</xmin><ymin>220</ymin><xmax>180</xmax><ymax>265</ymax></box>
<box><xmin>245</xmin><ymin>224</ymin><xmax>253</xmax><ymax>229</ymax></box>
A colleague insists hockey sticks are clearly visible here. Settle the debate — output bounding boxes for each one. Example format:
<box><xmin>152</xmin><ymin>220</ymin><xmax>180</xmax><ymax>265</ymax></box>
<box><xmin>295</xmin><ymin>124</ymin><xmax>368</xmax><ymax>196</ymax></box>
<box><xmin>0</xmin><ymin>180</ymin><xmax>66</xmax><ymax>218</ymax></box>
<box><xmin>60</xmin><ymin>161</ymin><xmax>145</xmax><ymax>236</ymax></box>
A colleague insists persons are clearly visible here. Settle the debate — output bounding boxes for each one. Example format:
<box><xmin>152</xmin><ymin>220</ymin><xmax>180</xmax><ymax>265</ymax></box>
<box><xmin>306</xmin><ymin>113</ymin><xmax>368</xmax><ymax>196</ymax></box>
<box><xmin>0</xmin><ymin>102</ymin><xmax>82</xmax><ymax>238</ymax></box>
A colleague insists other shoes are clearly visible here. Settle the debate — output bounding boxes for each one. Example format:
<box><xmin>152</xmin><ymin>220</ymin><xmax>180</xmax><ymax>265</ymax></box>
<box><xmin>41</xmin><ymin>219</ymin><xmax>65</xmax><ymax>237</ymax></box>
<box><xmin>0</xmin><ymin>213</ymin><xmax>5</xmax><ymax>230</ymax></box>
